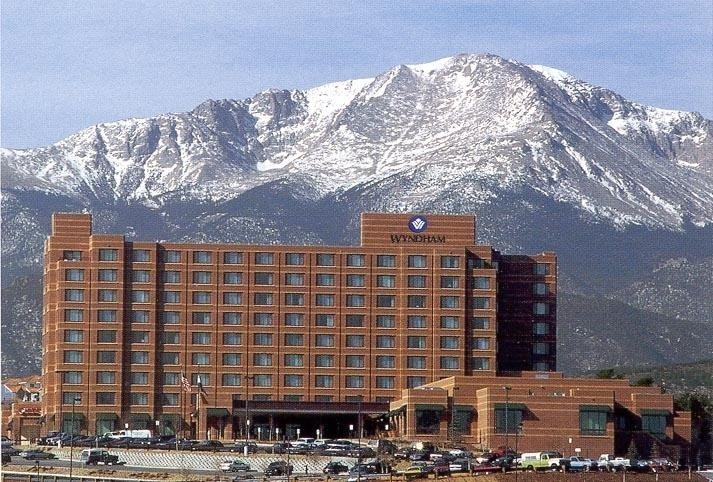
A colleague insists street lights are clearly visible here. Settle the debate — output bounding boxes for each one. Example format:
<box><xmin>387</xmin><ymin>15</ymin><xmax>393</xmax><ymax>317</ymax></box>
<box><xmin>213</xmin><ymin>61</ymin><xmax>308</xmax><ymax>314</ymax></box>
<box><xmin>503</xmin><ymin>384</ymin><xmax>512</xmax><ymax>459</ymax></box>
<box><xmin>69</xmin><ymin>398</ymin><xmax>82</xmax><ymax>482</ymax></box>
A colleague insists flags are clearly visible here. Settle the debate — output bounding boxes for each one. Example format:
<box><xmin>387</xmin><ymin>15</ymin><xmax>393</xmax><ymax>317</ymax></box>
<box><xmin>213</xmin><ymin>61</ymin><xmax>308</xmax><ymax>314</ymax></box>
<box><xmin>182</xmin><ymin>375</ymin><xmax>192</xmax><ymax>392</ymax></box>
<box><xmin>197</xmin><ymin>375</ymin><xmax>203</xmax><ymax>391</ymax></box>
<box><xmin>199</xmin><ymin>390</ymin><xmax>208</xmax><ymax>403</ymax></box>
